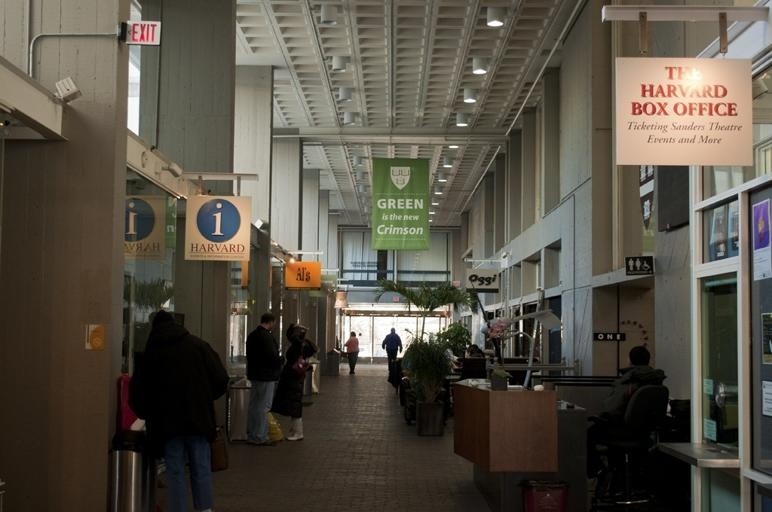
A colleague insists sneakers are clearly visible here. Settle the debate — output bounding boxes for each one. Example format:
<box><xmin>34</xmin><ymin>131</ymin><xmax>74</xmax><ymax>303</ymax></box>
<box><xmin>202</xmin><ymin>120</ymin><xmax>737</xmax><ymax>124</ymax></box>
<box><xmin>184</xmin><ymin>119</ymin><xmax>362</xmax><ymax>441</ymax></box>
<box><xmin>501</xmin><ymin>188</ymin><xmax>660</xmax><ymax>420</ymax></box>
<box><xmin>247</xmin><ymin>437</ymin><xmax>275</xmax><ymax>446</ymax></box>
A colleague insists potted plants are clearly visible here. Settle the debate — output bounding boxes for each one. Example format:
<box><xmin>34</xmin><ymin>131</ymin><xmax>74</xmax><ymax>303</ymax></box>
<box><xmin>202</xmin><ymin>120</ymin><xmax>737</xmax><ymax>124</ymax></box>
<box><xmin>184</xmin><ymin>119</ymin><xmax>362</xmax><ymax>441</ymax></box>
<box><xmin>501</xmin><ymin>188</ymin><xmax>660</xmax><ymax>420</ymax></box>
<box><xmin>403</xmin><ymin>339</ymin><xmax>455</xmax><ymax>437</ymax></box>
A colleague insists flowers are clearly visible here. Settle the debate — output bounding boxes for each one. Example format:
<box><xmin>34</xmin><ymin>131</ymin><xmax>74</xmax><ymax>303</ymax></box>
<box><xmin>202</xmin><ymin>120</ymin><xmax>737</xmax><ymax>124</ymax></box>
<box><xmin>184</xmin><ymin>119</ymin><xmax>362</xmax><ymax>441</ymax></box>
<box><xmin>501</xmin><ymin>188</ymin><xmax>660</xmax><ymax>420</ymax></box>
<box><xmin>481</xmin><ymin>316</ymin><xmax>510</xmax><ymax>347</ymax></box>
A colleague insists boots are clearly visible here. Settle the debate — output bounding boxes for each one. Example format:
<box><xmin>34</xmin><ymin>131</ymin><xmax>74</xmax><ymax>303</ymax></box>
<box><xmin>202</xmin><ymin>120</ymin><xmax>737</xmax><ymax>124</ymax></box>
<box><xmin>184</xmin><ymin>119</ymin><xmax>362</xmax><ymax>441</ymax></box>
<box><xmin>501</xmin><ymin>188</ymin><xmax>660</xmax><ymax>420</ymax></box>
<box><xmin>285</xmin><ymin>417</ymin><xmax>304</xmax><ymax>441</ymax></box>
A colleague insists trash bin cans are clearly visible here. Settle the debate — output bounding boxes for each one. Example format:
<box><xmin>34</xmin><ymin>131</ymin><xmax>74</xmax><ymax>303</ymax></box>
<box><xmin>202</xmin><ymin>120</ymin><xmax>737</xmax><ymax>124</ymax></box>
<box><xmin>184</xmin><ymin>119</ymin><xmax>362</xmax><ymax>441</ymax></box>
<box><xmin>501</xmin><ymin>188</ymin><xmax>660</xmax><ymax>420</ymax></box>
<box><xmin>327</xmin><ymin>348</ymin><xmax>341</xmax><ymax>376</ymax></box>
<box><xmin>111</xmin><ymin>430</ymin><xmax>157</xmax><ymax>512</ymax></box>
<box><xmin>303</xmin><ymin>360</ymin><xmax>321</xmax><ymax>396</ymax></box>
<box><xmin>517</xmin><ymin>479</ymin><xmax>571</xmax><ymax>512</ymax></box>
<box><xmin>227</xmin><ymin>378</ymin><xmax>253</xmax><ymax>443</ymax></box>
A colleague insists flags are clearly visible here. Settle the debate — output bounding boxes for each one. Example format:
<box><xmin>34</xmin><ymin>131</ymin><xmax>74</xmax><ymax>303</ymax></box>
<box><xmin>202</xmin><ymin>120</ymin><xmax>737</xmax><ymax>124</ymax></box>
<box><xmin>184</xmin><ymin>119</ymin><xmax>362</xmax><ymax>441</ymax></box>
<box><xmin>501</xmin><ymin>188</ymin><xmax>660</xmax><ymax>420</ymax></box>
<box><xmin>367</xmin><ymin>155</ymin><xmax>431</xmax><ymax>254</ymax></box>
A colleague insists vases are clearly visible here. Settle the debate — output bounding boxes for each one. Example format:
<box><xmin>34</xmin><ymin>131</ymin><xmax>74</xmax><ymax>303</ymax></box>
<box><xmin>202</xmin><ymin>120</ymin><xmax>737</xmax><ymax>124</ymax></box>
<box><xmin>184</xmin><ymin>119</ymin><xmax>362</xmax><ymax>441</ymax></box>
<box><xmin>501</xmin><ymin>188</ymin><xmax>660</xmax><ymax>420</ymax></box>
<box><xmin>488</xmin><ymin>348</ymin><xmax>503</xmax><ymax>383</ymax></box>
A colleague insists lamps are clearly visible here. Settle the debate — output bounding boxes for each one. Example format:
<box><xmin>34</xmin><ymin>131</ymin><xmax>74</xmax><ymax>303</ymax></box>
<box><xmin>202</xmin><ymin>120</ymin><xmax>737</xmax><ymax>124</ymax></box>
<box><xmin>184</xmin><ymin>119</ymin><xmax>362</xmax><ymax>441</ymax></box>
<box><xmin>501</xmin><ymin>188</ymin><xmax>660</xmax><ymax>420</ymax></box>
<box><xmin>53</xmin><ymin>77</ymin><xmax>82</xmax><ymax>103</ymax></box>
<box><xmin>427</xmin><ymin>7</ymin><xmax>507</xmax><ymax>223</ymax></box>
<box><xmin>162</xmin><ymin>162</ymin><xmax>182</xmax><ymax>177</ymax></box>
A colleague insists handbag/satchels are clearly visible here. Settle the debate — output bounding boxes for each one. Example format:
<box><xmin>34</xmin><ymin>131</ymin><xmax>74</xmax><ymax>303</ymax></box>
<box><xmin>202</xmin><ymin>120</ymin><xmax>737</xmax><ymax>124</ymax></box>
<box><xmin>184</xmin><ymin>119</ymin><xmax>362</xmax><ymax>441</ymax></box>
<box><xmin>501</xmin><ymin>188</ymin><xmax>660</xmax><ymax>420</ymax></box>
<box><xmin>211</xmin><ymin>435</ymin><xmax>228</xmax><ymax>473</ymax></box>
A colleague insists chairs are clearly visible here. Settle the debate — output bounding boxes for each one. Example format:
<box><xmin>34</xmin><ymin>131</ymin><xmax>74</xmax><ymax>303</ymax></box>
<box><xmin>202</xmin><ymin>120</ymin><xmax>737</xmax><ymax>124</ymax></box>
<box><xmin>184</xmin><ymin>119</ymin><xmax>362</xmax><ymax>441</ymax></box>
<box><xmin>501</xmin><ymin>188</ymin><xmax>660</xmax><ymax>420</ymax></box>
<box><xmin>586</xmin><ymin>384</ymin><xmax>669</xmax><ymax>505</ymax></box>
<box><xmin>457</xmin><ymin>357</ymin><xmax>487</xmax><ymax>380</ymax></box>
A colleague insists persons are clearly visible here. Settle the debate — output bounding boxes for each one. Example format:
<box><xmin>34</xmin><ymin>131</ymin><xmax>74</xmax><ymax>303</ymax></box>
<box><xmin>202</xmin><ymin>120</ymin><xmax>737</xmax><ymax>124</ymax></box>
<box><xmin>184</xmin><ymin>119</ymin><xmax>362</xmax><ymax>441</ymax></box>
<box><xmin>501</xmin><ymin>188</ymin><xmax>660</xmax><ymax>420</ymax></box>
<box><xmin>270</xmin><ymin>325</ymin><xmax>321</xmax><ymax>443</ymax></box>
<box><xmin>128</xmin><ymin>308</ymin><xmax>229</xmax><ymax>512</ymax></box>
<box><xmin>246</xmin><ymin>312</ymin><xmax>288</xmax><ymax>447</ymax></box>
<box><xmin>585</xmin><ymin>346</ymin><xmax>667</xmax><ymax>483</ymax></box>
<box><xmin>344</xmin><ymin>331</ymin><xmax>360</xmax><ymax>374</ymax></box>
<box><xmin>382</xmin><ymin>328</ymin><xmax>403</xmax><ymax>371</ymax></box>
<box><xmin>466</xmin><ymin>344</ymin><xmax>485</xmax><ymax>358</ymax></box>
